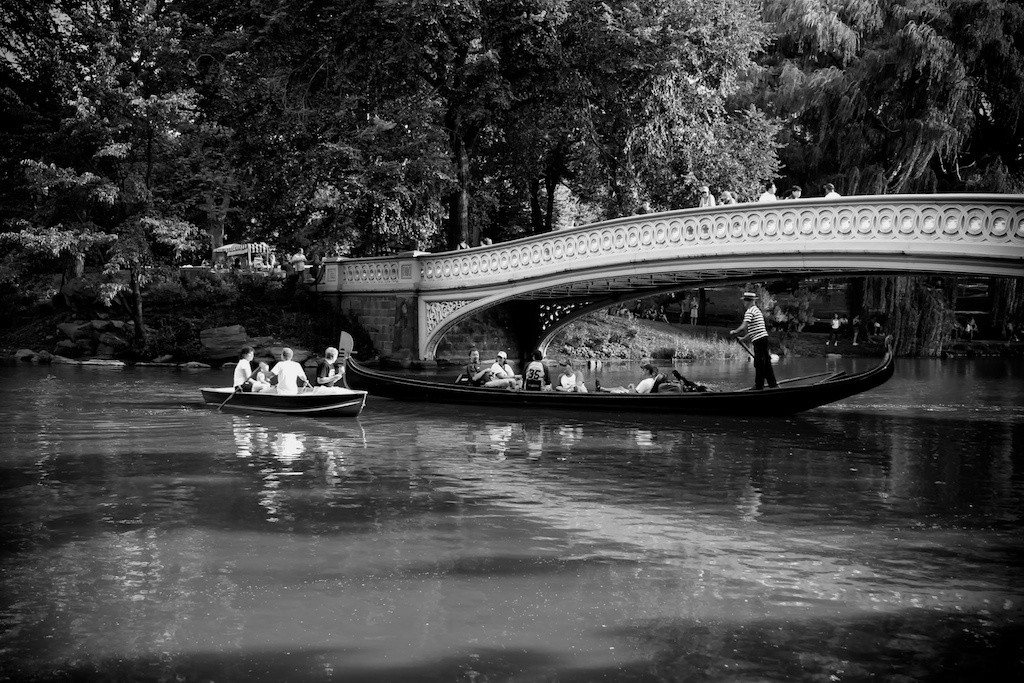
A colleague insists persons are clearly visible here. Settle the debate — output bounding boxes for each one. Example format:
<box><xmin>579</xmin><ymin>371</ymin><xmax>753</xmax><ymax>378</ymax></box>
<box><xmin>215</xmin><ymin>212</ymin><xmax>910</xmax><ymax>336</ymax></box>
<box><xmin>233</xmin><ymin>248</ymin><xmax>307</xmax><ymax>279</ymax></box>
<box><xmin>788</xmin><ymin>307</ymin><xmax>1020</xmax><ymax>345</ymax></box>
<box><xmin>480</xmin><ymin>238</ymin><xmax>492</xmax><ymax>246</ymax></box>
<box><xmin>786</xmin><ymin>186</ymin><xmax>802</xmax><ymax>199</ymax></box>
<box><xmin>759</xmin><ymin>181</ymin><xmax>777</xmax><ymax>201</ymax></box>
<box><xmin>233</xmin><ymin>347</ymin><xmax>270</xmax><ymax>393</ymax></box>
<box><xmin>259</xmin><ymin>348</ymin><xmax>309</xmax><ymax>394</ymax></box>
<box><xmin>257</xmin><ymin>364</ymin><xmax>271</xmax><ymax>385</ymax></box>
<box><xmin>730</xmin><ymin>292</ymin><xmax>779</xmax><ymax>389</ymax></box>
<box><xmin>313</xmin><ymin>347</ymin><xmax>345</xmax><ymax>388</ymax></box>
<box><xmin>457</xmin><ymin>237</ymin><xmax>470</xmax><ymax>250</ymax></box>
<box><xmin>631</xmin><ymin>198</ymin><xmax>655</xmax><ymax>215</ymax></box>
<box><xmin>699</xmin><ymin>186</ymin><xmax>738</xmax><ymax>207</ymax></box>
<box><xmin>823</xmin><ymin>183</ymin><xmax>841</xmax><ymax>196</ymax></box>
<box><xmin>608</xmin><ymin>294</ymin><xmax>699</xmax><ymax>326</ymax></box>
<box><xmin>466</xmin><ymin>347</ymin><xmax>658</xmax><ymax>393</ymax></box>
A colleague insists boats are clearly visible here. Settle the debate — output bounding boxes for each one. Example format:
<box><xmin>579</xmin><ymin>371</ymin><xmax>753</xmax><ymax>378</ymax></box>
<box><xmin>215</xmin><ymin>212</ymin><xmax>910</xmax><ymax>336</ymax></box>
<box><xmin>197</xmin><ymin>385</ymin><xmax>368</xmax><ymax>420</ymax></box>
<box><xmin>342</xmin><ymin>336</ymin><xmax>894</xmax><ymax>418</ymax></box>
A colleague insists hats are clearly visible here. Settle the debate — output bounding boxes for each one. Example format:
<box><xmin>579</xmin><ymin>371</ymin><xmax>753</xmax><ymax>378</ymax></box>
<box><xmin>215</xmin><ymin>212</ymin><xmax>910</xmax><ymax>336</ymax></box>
<box><xmin>497</xmin><ymin>351</ymin><xmax>507</xmax><ymax>359</ymax></box>
<box><xmin>559</xmin><ymin>359</ymin><xmax>571</xmax><ymax>366</ymax></box>
<box><xmin>740</xmin><ymin>292</ymin><xmax>759</xmax><ymax>300</ymax></box>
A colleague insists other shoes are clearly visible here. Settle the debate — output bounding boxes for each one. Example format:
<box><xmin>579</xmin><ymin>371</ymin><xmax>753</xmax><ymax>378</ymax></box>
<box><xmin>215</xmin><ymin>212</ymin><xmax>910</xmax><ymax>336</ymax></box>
<box><xmin>749</xmin><ymin>387</ymin><xmax>763</xmax><ymax>390</ymax></box>
<box><xmin>834</xmin><ymin>343</ymin><xmax>837</xmax><ymax>346</ymax></box>
<box><xmin>826</xmin><ymin>341</ymin><xmax>829</xmax><ymax>345</ymax></box>
<box><xmin>853</xmin><ymin>343</ymin><xmax>859</xmax><ymax>345</ymax></box>
